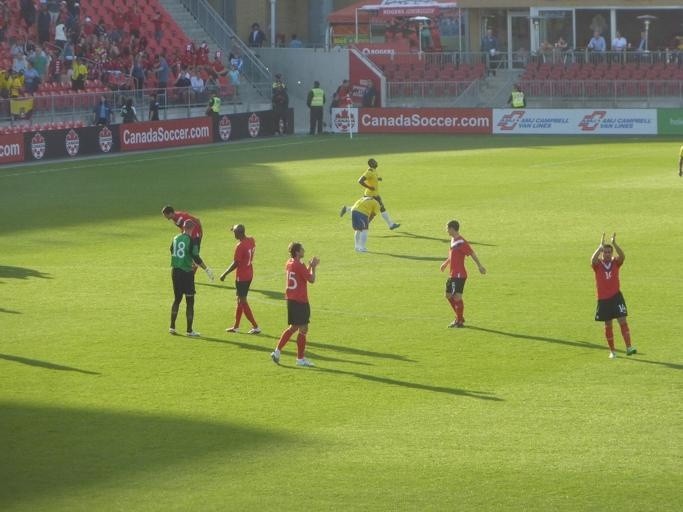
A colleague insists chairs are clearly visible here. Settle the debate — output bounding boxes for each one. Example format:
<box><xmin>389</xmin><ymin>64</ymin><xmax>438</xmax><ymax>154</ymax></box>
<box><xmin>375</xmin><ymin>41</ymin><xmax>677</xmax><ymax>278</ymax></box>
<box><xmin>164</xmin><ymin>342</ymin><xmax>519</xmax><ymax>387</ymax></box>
<box><xmin>1</xmin><ymin>0</ymin><xmax>240</xmax><ymax>114</ymax></box>
<box><xmin>516</xmin><ymin>60</ymin><xmax>683</xmax><ymax>97</ymax></box>
<box><xmin>379</xmin><ymin>56</ymin><xmax>487</xmax><ymax>97</ymax></box>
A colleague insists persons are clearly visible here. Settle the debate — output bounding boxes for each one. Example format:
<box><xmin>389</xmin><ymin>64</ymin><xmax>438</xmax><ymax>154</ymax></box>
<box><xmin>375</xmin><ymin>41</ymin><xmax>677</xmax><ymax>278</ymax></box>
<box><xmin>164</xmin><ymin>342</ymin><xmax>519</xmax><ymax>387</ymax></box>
<box><xmin>508</xmin><ymin>84</ymin><xmax>526</xmax><ymax>108</ymax></box>
<box><xmin>120</xmin><ymin>99</ymin><xmax>139</xmax><ymax>124</ymax></box>
<box><xmin>439</xmin><ymin>219</ymin><xmax>486</xmax><ymax>328</ymax></box>
<box><xmin>338</xmin><ymin>79</ymin><xmax>350</xmax><ymax>108</ymax></box>
<box><xmin>289</xmin><ymin>34</ymin><xmax>302</xmax><ymax>49</ymax></box>
<box><xmin>636</xmin><ymin>31</ymin><xmax>647</xmax><ymax>51</ymax></box>
<box><xmin>614</xmin><ymin>31</ymin><xmax>626</xmax><ymax>62</ymax></box>
<box><xmin>481</xmin><ymin>26</ymin><xmax>498</xmax><ymax>65</ymax></box>
<box><xmin>270</xmin><ymin>240</ymin><xmax>320</xmax><ymax>367</ymax></box>
<box><xmin>0</xmin><ymin>1</ymin><xmax>244</xmax><ymax>98</ymax></box>
<box><xmin>149</xmin><ymin>93</ymin><xmax>159</xmax><ymax>121</ymax></box>
<box><xmin>363</xmin><ymin>78</ymin><xmax>376</xmax><ymax>106</ymax></box>
<box><xmin>307</xmin><ymin>81</ymin><xmax>326</xmax><ymax>135</ymax></box>
<box><xmin>249</xmin><ymin>23</ymin><xmax>265</xmax><ymax>48</ymax></box>
<box><xmin>678</xmin><ymin>145</ymin><xmax>683</xmax><ymax>179</ymax></box>
<box><xmin>589</xmin><ymin>30</ymin><xmax>605</xmax><ymax>62</ymax></box>
<box><xmin>207</xmin><ymin>90</ymin><xmax>221</xmax><ymax>116</ymax></box>
<box><xmin>589</xmin><ymin>230</ymin><xmax>637</xmax><ymax>359</ymax></box>
<box><xmin>541</xmin><ymin>36</ymin><xmax>569</xmax><ymax>56</ymax></box>
<box><xmin>94</xmin><ymin>94</ymin><xmax>112</xmax><ymax>127</ymax></box>
<box><xmin>160</xmin><ymin>205</ymin><xmax>203</xmax><ymax>275</ymax></box>
<box><xmin>339</xmin><ymin>158</ymin><xmax>400</xmax><ymax>231</ymax></box>
<box><xmin>272</xmin><ymin>73</ymin><xmax>289</xmax><ymax>136</ymax></box>
<box><xmin>349</xmin><ymin>195</ymin><xmax>382</xmax><ymax>253</ymax></box>
<box><xmin>168</xmin><ymin>219</ymin><xmax>215</xmax><ymax>339</ymax></box>
<box><xmin>218</xmin><ymin>222</ymin><xmax>262</xmax><ymax>334</ymax></box>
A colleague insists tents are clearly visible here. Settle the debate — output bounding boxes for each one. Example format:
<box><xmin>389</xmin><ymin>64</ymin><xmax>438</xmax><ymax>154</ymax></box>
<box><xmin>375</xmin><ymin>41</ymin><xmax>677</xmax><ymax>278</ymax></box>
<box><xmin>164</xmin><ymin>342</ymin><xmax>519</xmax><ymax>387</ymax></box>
<box><xmin>327</xmin><ymin>0</ymin><xmax>461</xmax><ymax>53</ymax></box>
